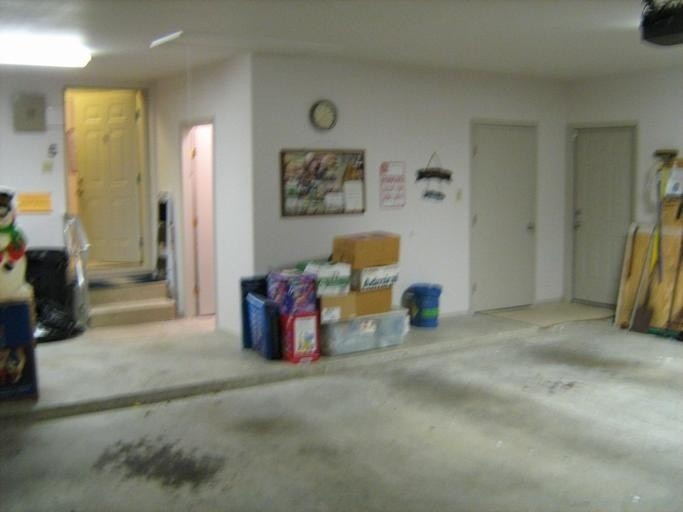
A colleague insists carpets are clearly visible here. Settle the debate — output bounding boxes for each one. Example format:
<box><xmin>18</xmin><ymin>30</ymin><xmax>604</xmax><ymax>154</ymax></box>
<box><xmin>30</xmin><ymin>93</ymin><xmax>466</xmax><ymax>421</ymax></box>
<box><xmin>480</xmin><ymin>302</ymin><xmax>615</xmax><ymax>329</ymax></box>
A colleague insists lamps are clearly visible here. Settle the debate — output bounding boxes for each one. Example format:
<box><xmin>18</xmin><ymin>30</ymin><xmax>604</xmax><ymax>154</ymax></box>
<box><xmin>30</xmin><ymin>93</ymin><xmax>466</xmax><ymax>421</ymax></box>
<box><xmin>640</xmin><ymin>0</ymin><xmax>682</xmax><ymax>46</ymax></box>
<box><xmin>0</xmin><ymin>27</ymin><xmax>93</xmax><ymax>68</ymax></box>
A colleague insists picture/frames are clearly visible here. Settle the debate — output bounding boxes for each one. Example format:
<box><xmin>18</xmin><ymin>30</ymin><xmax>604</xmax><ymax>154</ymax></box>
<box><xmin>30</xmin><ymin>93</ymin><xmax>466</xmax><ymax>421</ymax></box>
<box><xmin>280</xmin><ymin>148</ymin><xmax>366</xmax><ymax>216</ymax></box>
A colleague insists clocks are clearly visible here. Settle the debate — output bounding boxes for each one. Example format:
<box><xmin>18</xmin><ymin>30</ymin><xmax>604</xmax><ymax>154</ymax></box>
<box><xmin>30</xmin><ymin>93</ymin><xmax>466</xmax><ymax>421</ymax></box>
<box><xmin>310</xmin><ymin>99</ymin><xmax>337</xmax><ymax>129</ymax></box>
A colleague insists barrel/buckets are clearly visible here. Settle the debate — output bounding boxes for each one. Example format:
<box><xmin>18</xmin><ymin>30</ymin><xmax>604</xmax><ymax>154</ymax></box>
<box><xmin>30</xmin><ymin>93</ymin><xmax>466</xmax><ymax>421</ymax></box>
<box><xmin>406</xmin><ymin>283</ymin><xmax>441</xmax><ymax>326</ymax></box>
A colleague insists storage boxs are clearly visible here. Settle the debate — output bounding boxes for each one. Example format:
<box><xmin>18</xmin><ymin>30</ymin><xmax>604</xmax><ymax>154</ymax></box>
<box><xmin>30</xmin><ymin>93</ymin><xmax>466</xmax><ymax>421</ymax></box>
<box><xmin>320</xmin><ymin>305</ymin><xmax>410</xmax><ymax>357</ymax></box>
<box><xmin>245</xmin><ymin>290</ymin><xmax>281</xmax><ymax>358</ymax></box>
<box><xmin>351</xmin><ymin>263</ymin><xmax>400</xmax><ymax>291</ymax></box>
<box><xmin>319</xmin><ymin>291</ymin><xmax>357</xmax><ymax>323</ymax></box>
<box><xmin>296</xmin><ymin>258</ymin><xmax>351</xmax><ymax>298</ymax></box>
<box><xmin>280</xmin><ymin>310</ymin><xmax>321</xmax><ymax>362</ymax></box>
<box><xmin>331</xmin><ymin>229</ymin><xmax>400</xmax><ymax>269</ymax></box>
<box><xmin>352</xmin><ymin>287</ymin><xmax>392</xmax><ymax>316</ymax></box>
